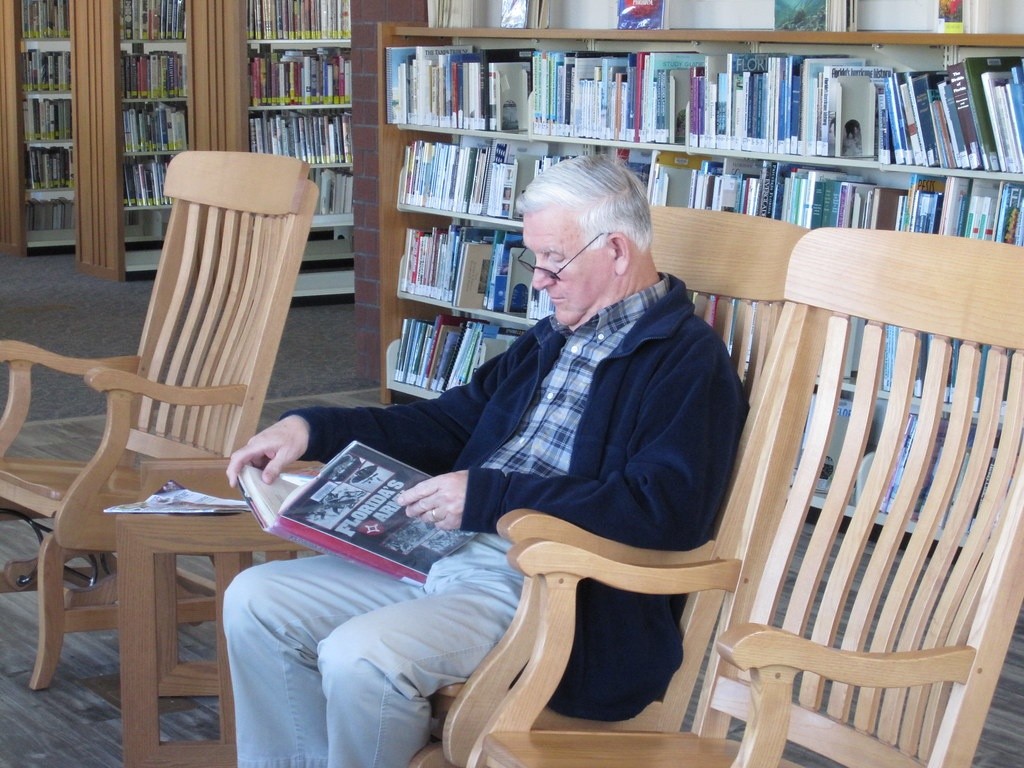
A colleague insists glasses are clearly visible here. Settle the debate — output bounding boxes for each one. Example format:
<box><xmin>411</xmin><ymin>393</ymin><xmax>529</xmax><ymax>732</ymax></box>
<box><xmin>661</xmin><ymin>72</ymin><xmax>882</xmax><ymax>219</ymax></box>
<box><xmin>517</xmin><ymin>231</ymin><xmax>614</xmax><ymax>280</ymax></box>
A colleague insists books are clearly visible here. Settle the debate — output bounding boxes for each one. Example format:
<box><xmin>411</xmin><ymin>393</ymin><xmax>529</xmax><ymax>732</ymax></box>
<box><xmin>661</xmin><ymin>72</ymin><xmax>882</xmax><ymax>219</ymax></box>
<box><xmin>120</xmin><ymin>0</ymin><xmax>187</xmax><ymax>226</ymax></box>
<box><xmin>20</xmin><ymin>0</ymin><xmax>76</xmax><ymax>229</ymax></box>
<box><xmin>103</xmin><ymin>480</ymin><xmax>251</xmax><ymax>514</ymax></box>
<box><xmin>247</xmin><ymin>0</ymin><xmax>352</xmax><ymax>213</ymax></box>
<box><xmin>390</xmin><ymin>0</ymin><xmax>1024</xmax><ymax>530</ymax></box>
<box><xmin>238</xmin><ymin>440</ymin><xmax>479</xmax><ymax>581</ymax></box>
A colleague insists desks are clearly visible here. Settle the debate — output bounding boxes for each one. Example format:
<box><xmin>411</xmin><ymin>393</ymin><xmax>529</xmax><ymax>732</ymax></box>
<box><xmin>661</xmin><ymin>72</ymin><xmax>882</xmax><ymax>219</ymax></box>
<box><xmin>114</xmin><ymin>459</ymin><xmax>326</xmax><ymax>768</ymax></box>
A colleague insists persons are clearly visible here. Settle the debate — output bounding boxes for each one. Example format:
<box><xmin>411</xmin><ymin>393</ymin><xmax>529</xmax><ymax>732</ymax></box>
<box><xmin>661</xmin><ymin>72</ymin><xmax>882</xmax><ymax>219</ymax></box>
<box><xmin>222</xmin><ymin>158</ymin><xmax>749</xmax><ymax>768</ymax></box>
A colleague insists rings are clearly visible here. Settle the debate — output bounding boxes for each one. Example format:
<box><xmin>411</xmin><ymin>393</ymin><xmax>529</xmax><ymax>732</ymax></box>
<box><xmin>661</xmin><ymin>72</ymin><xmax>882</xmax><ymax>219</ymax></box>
<box><xmin>432</xmin><ymin>510</ymin><xmax>440</xmax><ymax>521</ymax></box>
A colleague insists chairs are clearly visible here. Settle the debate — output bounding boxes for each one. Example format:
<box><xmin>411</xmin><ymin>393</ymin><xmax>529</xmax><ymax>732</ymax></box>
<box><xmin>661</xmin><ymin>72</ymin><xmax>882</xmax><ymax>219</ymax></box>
<box><xmin>466</xmin><ymin>228</ymin><xmax>1024</xmax><ymax>768</ymax></box>
<box><xmin>0</xmin><ymin>151</ymin><xmax>319</xmax><ymax>689</ymax></box>
<box><xmin>257</xmin><ymin>204</ymin><xmax>812</xmax><ymax>768</ymax></box>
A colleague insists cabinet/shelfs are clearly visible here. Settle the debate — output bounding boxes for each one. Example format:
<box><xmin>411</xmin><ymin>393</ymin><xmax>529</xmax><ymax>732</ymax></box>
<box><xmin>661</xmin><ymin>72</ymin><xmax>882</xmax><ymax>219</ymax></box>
<box><xmin>376</xmin><ymin>21</ymin><xmax>1024</xmax><ymax>568</ymax></box>
<box><xmin>185</xmin><ymin>0</ymin><xmax>355</xmax><ymax>309</ymax></box>
<box><xmin>70</xmin><ymin>0</ymin><xmax>189</xmax><ymax>281</ymax></box>
<box><xmin>0</xmin><ymin>0</ymin><xmax>76</xmax><ymax>257</ymax></box>
